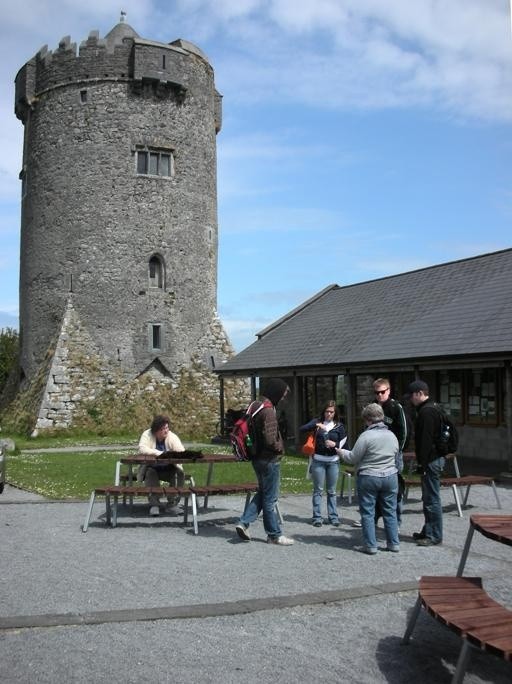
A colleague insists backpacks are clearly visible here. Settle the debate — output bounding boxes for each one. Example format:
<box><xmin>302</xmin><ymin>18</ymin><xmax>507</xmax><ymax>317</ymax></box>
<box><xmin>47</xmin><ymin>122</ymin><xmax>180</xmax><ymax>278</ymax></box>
<box><xmin>390</xmin><ymin>400</ymin><xmax>413</xmax><ymax>451</ymax></box>
<box><xmin>424</xmin><ymin>402</ymin><xmax>459</xmax><ymax>455</ymax></box>
<box><xmin>229</xmin><ymin>401</ymin><xmax>273</xmax><ymax>462</ymax></box>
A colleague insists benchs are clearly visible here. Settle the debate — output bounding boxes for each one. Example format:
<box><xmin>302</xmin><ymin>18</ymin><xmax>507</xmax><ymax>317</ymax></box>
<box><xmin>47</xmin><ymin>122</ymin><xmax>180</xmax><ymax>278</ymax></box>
<box><xmin>341</xmin><ymin>469</ymin><xmax>359</xmax><ymax>504</ymax></box>
<box><xmin>405</xmin><ymin>476</ymin><xmax>502</xmax><ymax>517</ymax></box>
<box><xmin>119</xmin><ymin>475</ymin><xmax>199</xmax><ymax>508</ymax></box>
<box><xmin>83</xmin><ymin>483</ymin><xmax>283</xmax><ymax>535</ymax></box>
<box><xmin>402</xmin><ymin>576</ymin><xmax>512</xmax><ymax>684</ymax></box>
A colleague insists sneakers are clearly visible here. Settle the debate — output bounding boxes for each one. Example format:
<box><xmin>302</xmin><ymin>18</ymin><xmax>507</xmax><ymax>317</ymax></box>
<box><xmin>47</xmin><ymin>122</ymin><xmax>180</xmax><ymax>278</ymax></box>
<box><xmin>353</xmin><ymin>545</ymin><xmax>376</xmax><ymax>554</ymax></box>
<box><xmin>353</xmin><ymin>520</ymin><xmax>362</xmax><ymax>527</ymax></box>
<box><xmin>166</xmin><ymin>507</ymin><xmax>184</xmax><ymax>515</ymax></box>
<box><xmin>380</xmin><ymin>544</ymin><xmax>399</xmax><ymax>552</ymax></box>
<box><xmin>235</xmin><ymin>524</ymin><xmax>250</xmax><ymax>540</ymax></box>
<box><xmin>150</xmin><ymin>507</ymin><xmax>159</xmax><ymax>516</ymax></box>
<box><xmin>413</xmin><ymin>531</ymin><xmax>434</xmax><ymax>546</ymax></box>
<box><xmin>268</xmin><ymin>536</ymin><xmax>293</xmax><ymax>545</ymax></box>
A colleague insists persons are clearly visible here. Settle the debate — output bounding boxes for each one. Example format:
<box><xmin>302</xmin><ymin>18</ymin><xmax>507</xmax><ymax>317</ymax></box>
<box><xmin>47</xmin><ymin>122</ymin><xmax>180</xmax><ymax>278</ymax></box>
<box><xmin>409</xmin><ymin>380</ymin><xmax>445</xmax><ymax>546</ymax></box>
<box><xmin>235</xmin><ymin>378</ymin><xmax>294</xmax><ymax>546</ymax></box>
<box><xmin>335</xmin><ymin>402</ymin><xmax>404</xmax><ymax>555</ymax></box>
<box><xmin>136</xmin><ymin>415</ymin><xmax>186</xmax><ymax>516</ymax></box>
<box><xmin>352</xmin><ymin>378</ymin><xmax>408</xmax><ymax>527</ymax></box>
<box><xmin>299</xmin><ymin>399</ymin><xmax>348</xmax><ymax>527</ymax></box>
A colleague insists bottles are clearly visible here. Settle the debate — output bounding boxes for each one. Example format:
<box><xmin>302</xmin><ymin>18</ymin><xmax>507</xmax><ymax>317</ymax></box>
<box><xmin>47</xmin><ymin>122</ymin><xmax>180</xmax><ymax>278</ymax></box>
<box><xmin>323</xmin><ymin>429</ymin><xmax>329</xmax><ymax>442</ymax></box>
<box><xmin>441</xmin><ymin>424</ymin><xmax>451</xmax><ymax>441</ymax></box>
<box><xmin>246</xmin><ymin>433</ymin><xmax>252</xmax><ymax>447</ymax></box>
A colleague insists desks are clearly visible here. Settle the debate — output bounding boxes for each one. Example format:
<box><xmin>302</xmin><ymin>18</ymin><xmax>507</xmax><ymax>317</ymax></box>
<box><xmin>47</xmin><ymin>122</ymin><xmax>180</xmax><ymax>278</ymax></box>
<box><xmin>112</xmin><ymin>454</ymin><xmax>283</xmax><ymax>527</ymax></box>
<box><xmin>403</xmin><ymin>452</ymin><xmax>465</xmax><ymax>504</ymax></box>
<box><xmin>456</xmin><ymin>514</ymin><xmax>512</xmax><ymax>577</ymax></box>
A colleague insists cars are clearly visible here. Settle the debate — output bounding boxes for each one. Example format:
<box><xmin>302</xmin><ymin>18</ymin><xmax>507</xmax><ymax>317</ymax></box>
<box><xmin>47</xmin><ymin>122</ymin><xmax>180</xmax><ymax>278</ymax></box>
<box><xmin>0</xmin><ymin>427</ymin><xmax>17</xmax><ymax>493</ymax></box>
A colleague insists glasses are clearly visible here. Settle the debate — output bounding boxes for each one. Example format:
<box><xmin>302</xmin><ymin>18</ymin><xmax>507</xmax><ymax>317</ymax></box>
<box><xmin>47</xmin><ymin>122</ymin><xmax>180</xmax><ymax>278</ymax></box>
<box><xmin>375</xmin><ymin>388</ymin><xmax>387</xmax><ymax>395</ymax></box>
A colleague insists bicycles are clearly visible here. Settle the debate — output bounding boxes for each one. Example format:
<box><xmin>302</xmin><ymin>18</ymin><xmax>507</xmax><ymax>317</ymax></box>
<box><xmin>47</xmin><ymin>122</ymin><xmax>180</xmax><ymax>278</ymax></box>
<box><xmin>215</xmin><ymin>407</ymin><xmax>247</xmax><ymax>439</ymax></box>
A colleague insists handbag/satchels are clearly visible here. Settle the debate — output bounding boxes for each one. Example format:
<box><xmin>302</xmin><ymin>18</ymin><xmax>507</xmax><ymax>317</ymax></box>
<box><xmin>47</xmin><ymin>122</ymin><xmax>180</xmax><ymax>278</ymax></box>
<box><xmin>302</xmin><ymin>431</ymin><xmax>315</xmax><ymax>455</ymax></box>
